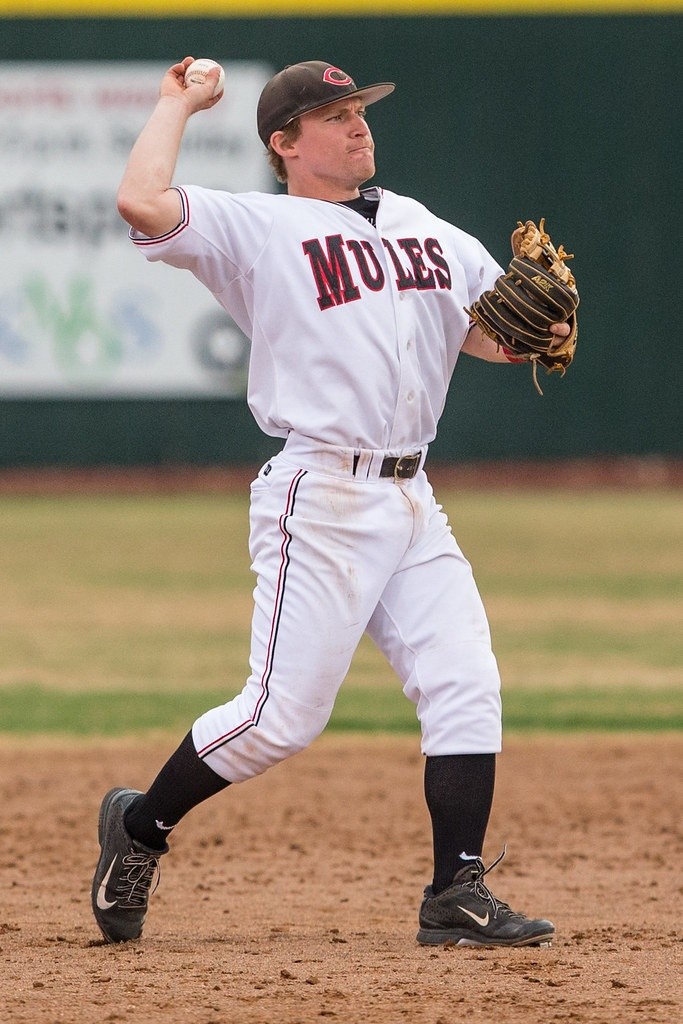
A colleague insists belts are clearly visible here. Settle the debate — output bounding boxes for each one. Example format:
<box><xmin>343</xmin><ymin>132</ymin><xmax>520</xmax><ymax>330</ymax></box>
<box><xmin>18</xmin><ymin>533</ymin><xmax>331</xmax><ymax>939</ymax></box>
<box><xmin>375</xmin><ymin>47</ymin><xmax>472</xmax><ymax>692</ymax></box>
<box><xmin>352</xmin><ymin>451</ymin><xmax>422</xmax><ymax>481</ymax></box>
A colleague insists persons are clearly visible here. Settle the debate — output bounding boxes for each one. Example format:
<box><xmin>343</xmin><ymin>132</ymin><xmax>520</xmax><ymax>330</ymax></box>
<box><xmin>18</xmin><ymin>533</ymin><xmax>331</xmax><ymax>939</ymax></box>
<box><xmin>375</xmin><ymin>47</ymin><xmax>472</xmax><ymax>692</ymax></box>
<box><xmin>90</xmin><ymin>57</ymin><xmax>581</xmax><ymax>947</ymax></box>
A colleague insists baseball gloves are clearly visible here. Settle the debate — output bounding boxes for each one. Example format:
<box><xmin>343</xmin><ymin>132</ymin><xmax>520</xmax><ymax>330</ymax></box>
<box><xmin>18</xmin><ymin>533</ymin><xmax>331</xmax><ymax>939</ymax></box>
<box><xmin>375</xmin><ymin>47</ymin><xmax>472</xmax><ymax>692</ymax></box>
<box><xmin>462</xmin><ymin>217</ymin><xmax>580</xmax><ymax>397</ymax></box>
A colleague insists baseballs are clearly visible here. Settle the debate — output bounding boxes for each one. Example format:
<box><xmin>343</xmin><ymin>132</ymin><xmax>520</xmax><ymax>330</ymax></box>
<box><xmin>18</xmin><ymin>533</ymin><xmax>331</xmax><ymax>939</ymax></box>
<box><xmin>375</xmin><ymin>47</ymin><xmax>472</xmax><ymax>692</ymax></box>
<box><xmin>184</xmin><ymin>57</ymin><xmax>225</xmax><ymax>99</ymax></box>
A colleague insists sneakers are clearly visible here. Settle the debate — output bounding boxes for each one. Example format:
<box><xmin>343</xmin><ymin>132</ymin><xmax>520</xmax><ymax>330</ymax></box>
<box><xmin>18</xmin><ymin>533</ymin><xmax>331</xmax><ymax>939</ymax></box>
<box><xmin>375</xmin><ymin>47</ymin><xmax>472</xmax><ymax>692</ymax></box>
<box><xmin>91</xmin><ymin>787</ymin><xmax>169</xmax><ymax>943</ymax></box>
<box><xmin>416</xmin><ymin>843</ymin><xmax>555</xmax><ymax>947</ymax></box>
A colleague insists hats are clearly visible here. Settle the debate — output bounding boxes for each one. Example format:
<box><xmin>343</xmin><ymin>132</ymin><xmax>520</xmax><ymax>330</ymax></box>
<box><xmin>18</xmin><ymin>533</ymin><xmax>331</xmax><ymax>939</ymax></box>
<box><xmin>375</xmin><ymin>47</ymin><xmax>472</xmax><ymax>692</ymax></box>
<box><xmin>257</xmin><ymin>60</ymin><xmax>396</xmax><ymax>151</ymax></box>
